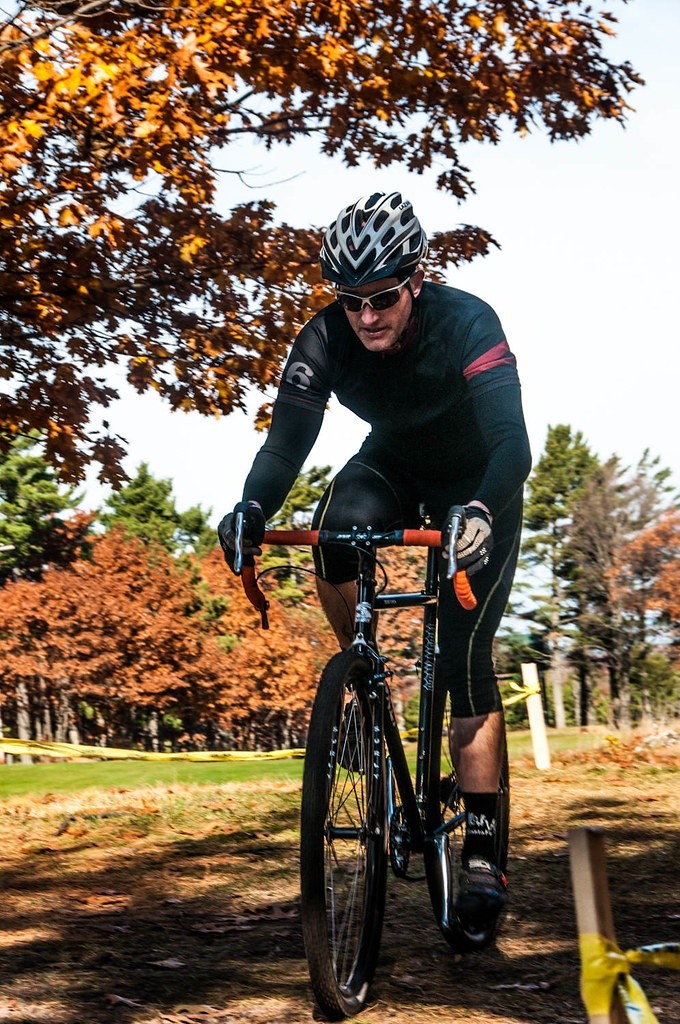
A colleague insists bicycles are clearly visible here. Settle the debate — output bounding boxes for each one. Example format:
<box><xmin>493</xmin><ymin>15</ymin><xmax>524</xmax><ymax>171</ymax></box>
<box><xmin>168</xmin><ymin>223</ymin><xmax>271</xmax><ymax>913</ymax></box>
<box><xmin>236</xmin><ymin>501</ymin><xmax>516</xmax><ymax>1022</ymax></box>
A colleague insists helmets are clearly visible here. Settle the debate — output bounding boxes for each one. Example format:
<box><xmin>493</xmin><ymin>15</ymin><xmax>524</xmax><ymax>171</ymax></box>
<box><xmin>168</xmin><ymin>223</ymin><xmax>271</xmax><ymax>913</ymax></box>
<box><xmin>320</xmin><ymin>191</ymin><xmax>428</xmax><ymax>288</ymax></box>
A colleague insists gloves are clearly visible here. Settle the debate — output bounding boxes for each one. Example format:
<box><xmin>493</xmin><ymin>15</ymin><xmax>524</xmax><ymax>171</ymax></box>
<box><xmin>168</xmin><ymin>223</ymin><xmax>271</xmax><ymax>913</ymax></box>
<box><xmin>217</xmin><ymin>500</ymin><xmax>265</xmax><ymax>577</ymax></box>
<box><xmin>441</xmin><ymin>501</ymin><xmax>492</xmax><ymax>577</ymax></box>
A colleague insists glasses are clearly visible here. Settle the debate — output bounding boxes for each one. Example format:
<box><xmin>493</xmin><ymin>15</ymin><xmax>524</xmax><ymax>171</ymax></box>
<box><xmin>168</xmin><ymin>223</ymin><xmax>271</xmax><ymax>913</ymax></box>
<box><xmin>334</xmin><ymin>271</ymin><xmax>416</xmax><ymax>313</ymax></box>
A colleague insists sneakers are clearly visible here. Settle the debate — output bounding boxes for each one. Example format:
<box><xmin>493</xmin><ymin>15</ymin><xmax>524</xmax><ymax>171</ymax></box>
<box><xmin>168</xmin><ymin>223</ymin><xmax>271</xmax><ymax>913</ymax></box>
<box><xmin>457</xmin><ymin>855</ymin><xmax>507</xmax><ymax>902</ymax></box>
<box><xmin>335</xmin><ymin>699</ymin><xmax>368</xmax><ymax>773</ymax></box>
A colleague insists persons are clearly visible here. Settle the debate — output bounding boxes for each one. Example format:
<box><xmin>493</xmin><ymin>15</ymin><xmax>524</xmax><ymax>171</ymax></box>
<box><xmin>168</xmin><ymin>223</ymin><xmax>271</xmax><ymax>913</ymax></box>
<box><xmin>216</xmin><ymin>191</ymin><xmax>533</xmax><ymax>899</ymax></box>
